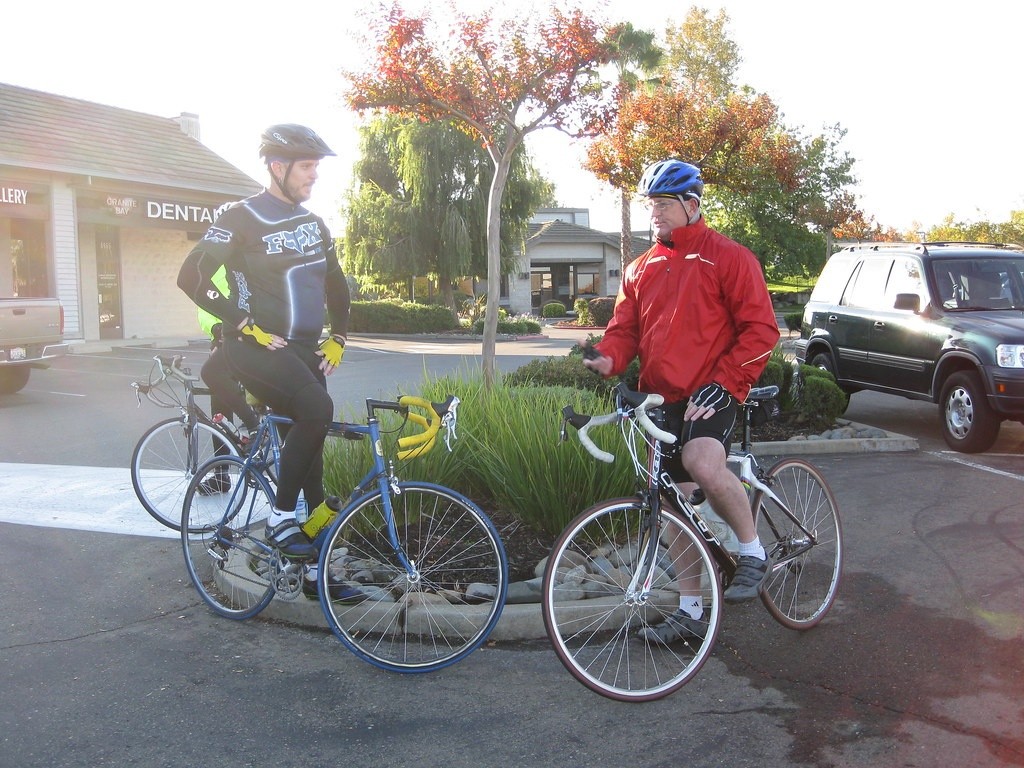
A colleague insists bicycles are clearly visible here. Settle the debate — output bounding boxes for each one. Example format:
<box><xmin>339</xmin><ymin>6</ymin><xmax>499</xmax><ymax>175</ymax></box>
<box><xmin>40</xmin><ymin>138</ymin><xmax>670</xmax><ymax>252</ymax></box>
<box><xmin>178</xmin><ymin>379</ymin><xmax>509</xmax><ymax>674</ymax></box>
<box><xmin>131</xmin><ymin>352</ymin><xmax>266</xmax><ymax>536</ymax></box>
<box><xmin>540</xmin><ymin>349</ymin><xmax>844</xmax><ymax>704</ymax></box>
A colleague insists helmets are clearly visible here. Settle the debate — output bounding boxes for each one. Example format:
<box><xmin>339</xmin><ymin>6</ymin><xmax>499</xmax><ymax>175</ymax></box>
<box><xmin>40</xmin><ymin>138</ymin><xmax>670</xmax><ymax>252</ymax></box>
<box><xmin>258</xmin><ymin>123</ymin><xmax>337</xmax><ymax>157</ymax></box>
<box><xmin>639</xmin><ymin>159</ymin><xmax>704</xmax><ymax>197</ymax></box>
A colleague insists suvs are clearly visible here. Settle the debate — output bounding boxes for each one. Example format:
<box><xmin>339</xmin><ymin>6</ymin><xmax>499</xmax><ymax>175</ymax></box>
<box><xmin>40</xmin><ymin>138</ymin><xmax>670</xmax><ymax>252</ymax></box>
<box><xmin>794</xmin><ymin>241</ymin><xmax>1023</xmax><ymax>455</ymax></box>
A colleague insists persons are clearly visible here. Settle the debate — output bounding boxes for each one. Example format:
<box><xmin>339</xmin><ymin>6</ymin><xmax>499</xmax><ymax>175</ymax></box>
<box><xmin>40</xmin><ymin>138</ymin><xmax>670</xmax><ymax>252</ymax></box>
<box><xmin>176</xmin><ymin>125</ymin><xmax>369</xmax><ymax>606</ymax></box>
<box><xmin>195</xmin><ymin>201</ymin><xmax>267</xmax><ymax>496</ymax></box>
<box><xmin>578</xmin><ymin>159</ymin><xmax>780</xmax><ymax>645</ymax></box>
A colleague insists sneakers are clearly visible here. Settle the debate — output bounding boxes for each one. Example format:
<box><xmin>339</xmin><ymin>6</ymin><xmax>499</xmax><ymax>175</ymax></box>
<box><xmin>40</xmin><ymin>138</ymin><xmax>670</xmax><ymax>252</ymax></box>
<box><xmin>302</xmin><ymin>570</ymin><xmax>365</xmax><ymax>604</ymax></box>
<box><xmin>638</xmin><ymin>608</ymin><xmax>708</xmax><ymax>644</ymax></box>
<box><xmin>248</xmin><ymin>477</ymin><xmax>256</xmax><ymax>487</ymax></box>
<box><xmin>265</xmin><ymin>518</ymin><xmax>319</xmax><ymax>559</ymax></box>
<box><xmin>724</xmin><ymin>551</ymin><xmax>773</xmax><ymax>601</ymax></box>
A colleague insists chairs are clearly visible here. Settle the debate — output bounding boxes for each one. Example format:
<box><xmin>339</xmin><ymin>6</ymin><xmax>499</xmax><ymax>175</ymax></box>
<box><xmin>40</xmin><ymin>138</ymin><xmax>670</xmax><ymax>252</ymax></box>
<box><xmin>963</xmin><ymin>270</ymin><xmax>1011</xmax><ymax>310</ymax></box>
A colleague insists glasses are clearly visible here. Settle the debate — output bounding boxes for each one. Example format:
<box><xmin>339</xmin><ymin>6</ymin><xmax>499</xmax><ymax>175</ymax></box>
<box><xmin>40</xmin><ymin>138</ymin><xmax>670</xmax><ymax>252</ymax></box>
<box><xmin>651</xmin><ymin>202</ymin><xmax>684</xmax><ymax>210</ymax></box>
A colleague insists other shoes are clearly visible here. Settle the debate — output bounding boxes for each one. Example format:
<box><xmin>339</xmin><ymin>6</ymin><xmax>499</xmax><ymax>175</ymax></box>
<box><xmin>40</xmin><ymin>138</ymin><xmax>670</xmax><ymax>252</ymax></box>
<box><xmin>196</xmin><ymin>475</ymin><xmax>231</xmax><ymax>495</ymax></box>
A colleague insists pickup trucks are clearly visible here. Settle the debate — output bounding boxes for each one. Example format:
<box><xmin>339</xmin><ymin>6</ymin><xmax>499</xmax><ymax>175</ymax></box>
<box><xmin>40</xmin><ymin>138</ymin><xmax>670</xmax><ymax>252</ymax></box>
<box><xmin>0</xmin><ymin>295</ymin><xmax>72</xmax><ymax>395</ymax></box>
